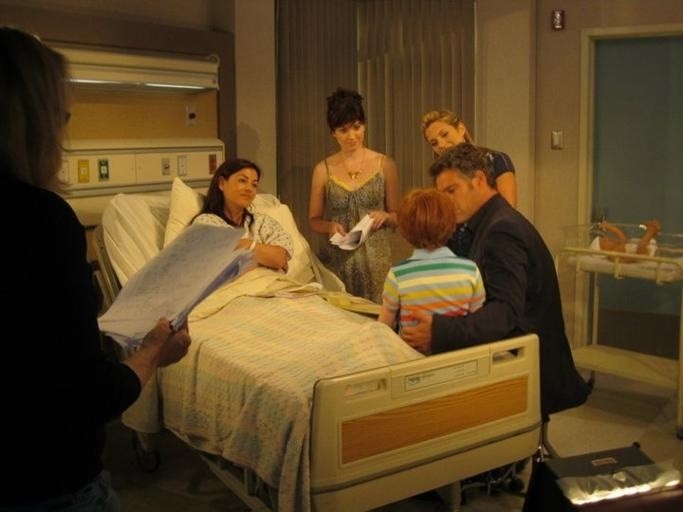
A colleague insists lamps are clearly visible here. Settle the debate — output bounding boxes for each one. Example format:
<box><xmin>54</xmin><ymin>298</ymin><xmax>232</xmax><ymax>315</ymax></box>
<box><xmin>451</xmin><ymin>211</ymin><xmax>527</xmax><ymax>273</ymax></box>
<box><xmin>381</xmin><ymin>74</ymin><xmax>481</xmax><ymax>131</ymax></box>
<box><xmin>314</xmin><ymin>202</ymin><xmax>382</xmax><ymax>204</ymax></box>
<box><xmin>551</xmin><ymin>9</ymin><xmax>564</xmax><ymax>31</ymax></box>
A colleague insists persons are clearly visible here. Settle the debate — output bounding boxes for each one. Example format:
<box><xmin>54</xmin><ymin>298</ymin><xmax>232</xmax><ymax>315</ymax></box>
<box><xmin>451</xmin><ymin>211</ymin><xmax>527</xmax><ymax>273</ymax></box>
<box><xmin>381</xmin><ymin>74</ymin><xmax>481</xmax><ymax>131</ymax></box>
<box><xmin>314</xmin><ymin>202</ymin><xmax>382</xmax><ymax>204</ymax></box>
<box><xmin>590</xmin><ymin>219</ymin><xmax>663</xmax><ymax>265</ymax></box>
<box><xmin>377</xmin><ymin>187</ymin><xmax>486</xmax><ymax>350</ymax></box>
<box><xmin>400</xmin><ymin>141</ymin><xmax>589</xmax><ymax>495</ymax></box>
<box><xmin>308</xmin><ymin>90</ymin><xmax>400</xmax><ymax>306</ymax></box>
<box><xmin>0</xmin><ymin>25</ymin><xmax>191</xmax><ymax>512</ymax></box>
<box><xmin>189</xmin><ymin>159</ymin><xmax>293</xmax><ymax>273</ymax></box>
<box><xmin>421</xmin><ymin>108</ymin><xmax>517</xmax><ymax>209</ymax></box>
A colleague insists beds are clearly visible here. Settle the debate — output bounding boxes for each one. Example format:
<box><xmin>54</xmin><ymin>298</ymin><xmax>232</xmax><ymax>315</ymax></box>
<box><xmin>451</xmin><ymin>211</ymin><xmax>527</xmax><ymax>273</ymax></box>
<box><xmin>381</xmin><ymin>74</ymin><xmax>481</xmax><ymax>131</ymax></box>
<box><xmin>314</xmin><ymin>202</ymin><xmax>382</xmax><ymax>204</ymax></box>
<box><xmin>90</xmin><ymin>185</ymin><xmax>541</xmax><ymax>512</ymax></box>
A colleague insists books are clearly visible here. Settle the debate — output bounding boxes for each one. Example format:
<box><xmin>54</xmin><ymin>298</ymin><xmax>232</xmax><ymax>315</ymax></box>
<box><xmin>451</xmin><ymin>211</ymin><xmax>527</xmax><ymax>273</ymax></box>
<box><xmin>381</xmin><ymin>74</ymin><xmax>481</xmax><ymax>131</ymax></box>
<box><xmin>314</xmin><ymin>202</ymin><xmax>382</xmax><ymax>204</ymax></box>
<box><xmin>96</xmin><ymin>222</ymin><xmax>248</xmax><ymax>351</ymax></box>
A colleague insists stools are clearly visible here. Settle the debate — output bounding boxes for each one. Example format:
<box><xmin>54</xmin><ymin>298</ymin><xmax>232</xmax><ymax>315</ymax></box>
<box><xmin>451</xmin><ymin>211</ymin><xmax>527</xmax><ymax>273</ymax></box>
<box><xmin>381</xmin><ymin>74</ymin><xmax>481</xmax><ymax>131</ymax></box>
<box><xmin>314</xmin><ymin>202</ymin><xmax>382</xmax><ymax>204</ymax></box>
<box><xmin>457</xmin><ymin>376</ymin><xmax>588</xmax><ymax>504</ymax></box>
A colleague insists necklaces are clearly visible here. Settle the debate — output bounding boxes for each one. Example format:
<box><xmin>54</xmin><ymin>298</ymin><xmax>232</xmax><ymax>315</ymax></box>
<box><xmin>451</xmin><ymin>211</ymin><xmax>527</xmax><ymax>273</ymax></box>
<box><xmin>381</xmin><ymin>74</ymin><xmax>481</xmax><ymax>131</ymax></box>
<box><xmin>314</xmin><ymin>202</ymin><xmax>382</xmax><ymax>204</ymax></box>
<box><xmin>341</xmin><ymin>148</ymin><xmax>366</xmax><ymax>180</ymax></box>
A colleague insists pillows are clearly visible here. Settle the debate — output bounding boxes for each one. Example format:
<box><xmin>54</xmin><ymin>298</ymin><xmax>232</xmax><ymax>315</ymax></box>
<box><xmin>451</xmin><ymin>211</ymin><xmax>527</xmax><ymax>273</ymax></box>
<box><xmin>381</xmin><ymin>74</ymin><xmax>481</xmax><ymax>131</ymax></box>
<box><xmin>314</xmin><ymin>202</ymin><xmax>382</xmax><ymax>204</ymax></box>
<box><xmin>164</xmin><ymin>177</ymin><xmax>311</xmax><ymax>282</ymax></box>
